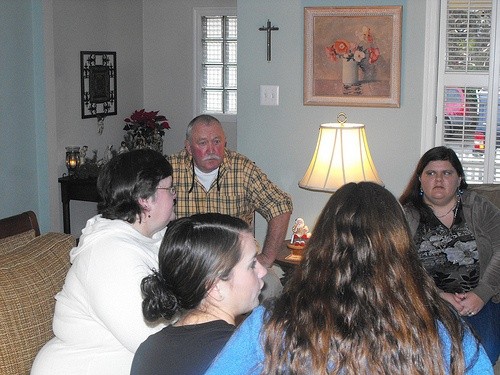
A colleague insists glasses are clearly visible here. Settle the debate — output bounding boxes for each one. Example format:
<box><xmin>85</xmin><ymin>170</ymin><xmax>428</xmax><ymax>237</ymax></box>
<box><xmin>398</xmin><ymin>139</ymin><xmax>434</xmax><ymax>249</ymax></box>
<box><xmin>155</xmin><ymin>185</ymin><xmax>176</xmax><ymax>195</ymax></box>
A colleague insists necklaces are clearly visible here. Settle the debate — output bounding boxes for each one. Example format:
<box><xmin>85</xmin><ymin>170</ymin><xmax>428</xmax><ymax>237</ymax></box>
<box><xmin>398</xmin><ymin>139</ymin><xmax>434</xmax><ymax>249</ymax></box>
<box><xmin>424</xmin><ymin>194</ymin><xmax>461</xmax><ymax>219</ymax></box>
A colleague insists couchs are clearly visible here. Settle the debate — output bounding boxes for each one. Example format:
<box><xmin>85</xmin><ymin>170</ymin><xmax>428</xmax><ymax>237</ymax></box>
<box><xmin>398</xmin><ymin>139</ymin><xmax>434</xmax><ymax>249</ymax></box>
<box><xmin>468</xmin><ymin>183</ymin><xmax>500</xmax><ymax>375</ymax></box>
<box><xmin>0</xmin><ymin>210</ymin><xmax>77</xmax><ymax>375</ymax></box>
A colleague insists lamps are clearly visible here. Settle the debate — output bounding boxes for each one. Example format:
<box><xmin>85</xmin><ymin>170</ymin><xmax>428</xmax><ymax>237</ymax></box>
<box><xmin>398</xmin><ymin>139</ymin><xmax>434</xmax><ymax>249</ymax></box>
<box><xmin>65</xmin><ymin>146</ymin><xmax>81</xmax><ymax>176</ymax></box>
<box><xmin>297</xmin><ymin>112</ymin><xmax>385</xmax><ymax>198</ymax></box>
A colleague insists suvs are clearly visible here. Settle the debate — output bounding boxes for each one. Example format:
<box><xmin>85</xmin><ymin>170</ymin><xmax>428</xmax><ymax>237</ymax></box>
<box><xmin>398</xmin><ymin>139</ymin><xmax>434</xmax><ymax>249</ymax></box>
<box><xmin>443</xmin><ymin>86</ymin><xmax>471</xmax><ymax>140</ymax></box>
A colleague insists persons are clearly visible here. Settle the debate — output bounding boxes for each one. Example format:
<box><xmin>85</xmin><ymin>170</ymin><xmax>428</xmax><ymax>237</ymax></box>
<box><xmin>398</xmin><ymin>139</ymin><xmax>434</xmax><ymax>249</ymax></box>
<box><xmin>203</xmin><ymin>181</ymin><xmax>494</xmax><ymax>375</ymax></box>
<box><xmin>398</xmin><ymin>146</ymin><xmax>500</xmax><ymax>364</ymax></box>
<box><xmin>165</xmin><ymin>114</ymin><xmax>293</xmax><ymax>302</ymax></box>
<box><xmin>130</xmin><ymin>212</ymin><xmax>267</xmax><ymax>375</ymax></box>
<box><xmin>30</xmin><ymin>148</ymin><xmax>177</xmax><ymax>375</ymax></box>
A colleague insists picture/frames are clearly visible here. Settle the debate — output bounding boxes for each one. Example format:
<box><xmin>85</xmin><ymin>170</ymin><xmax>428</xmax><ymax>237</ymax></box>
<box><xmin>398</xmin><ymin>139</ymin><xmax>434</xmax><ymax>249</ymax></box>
<box><xmin>304</xmin><ymin>5</ymin><xmax>403</xmax><ymax>109</ymax></box>
<box><xmin>80</xmin><ymin>50</ymin><xmax>118</xmax><ymax>120</ymax></box>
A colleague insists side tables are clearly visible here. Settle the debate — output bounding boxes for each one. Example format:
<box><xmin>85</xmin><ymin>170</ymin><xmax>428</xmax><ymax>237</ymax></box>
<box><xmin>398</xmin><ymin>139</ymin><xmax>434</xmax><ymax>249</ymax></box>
<box><xmin>274</xmin><ymin>238</ymin><xmax>310</xmax><ymax>286</ymax></box>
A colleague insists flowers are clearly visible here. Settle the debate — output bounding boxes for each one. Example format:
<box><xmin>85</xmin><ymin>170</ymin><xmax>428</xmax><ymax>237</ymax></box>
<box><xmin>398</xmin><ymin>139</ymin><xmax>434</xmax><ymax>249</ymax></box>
<box><xmin>123</xmin><ymin>108</ymin><xmax>171</xmax><ymax>146</ymax></box>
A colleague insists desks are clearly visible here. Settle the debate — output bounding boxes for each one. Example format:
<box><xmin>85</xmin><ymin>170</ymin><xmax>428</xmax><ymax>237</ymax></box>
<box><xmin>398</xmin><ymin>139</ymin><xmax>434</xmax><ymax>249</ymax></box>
<box><xmin>58</xmin><ymin>175</ymin><xmax>98</xmax><ymax>234</ymax></box>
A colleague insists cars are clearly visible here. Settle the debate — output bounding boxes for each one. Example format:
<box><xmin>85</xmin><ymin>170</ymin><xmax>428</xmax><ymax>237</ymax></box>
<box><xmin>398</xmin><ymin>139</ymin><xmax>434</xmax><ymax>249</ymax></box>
<box><xmin>472</xmin><ymin>97</ymin><xmax>500</xmax><ymax>157</ymax></box>
